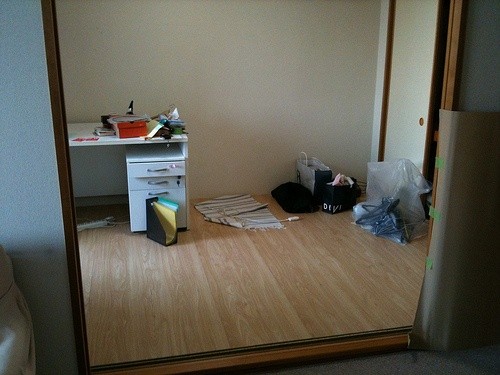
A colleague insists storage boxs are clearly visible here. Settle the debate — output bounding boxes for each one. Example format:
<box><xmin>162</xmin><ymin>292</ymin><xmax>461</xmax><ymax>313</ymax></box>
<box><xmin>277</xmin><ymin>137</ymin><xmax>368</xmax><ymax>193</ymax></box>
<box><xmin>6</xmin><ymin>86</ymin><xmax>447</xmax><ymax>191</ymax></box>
<box><xmin>114</xmin><ymin>121</ymin><xmax>147</xmax><ymax>139</ymax></box>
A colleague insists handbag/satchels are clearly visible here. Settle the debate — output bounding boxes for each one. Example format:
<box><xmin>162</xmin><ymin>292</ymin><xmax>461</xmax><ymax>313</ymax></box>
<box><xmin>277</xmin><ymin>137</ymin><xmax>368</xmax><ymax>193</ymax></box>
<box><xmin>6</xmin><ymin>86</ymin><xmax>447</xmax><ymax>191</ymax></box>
<box><xmin>272</xmin><ymin>182</ymin><xmax>312</xmax><ymax>213</ymax></box>
<box><xmin>321</xmin><ymin>177</ymin><xmax>357</xmax><ymax>215</ymax></box>
<box><xmin>297</xmin><ymin>152</ymin><xmax>332</xmax><ymax>208</ymax></box>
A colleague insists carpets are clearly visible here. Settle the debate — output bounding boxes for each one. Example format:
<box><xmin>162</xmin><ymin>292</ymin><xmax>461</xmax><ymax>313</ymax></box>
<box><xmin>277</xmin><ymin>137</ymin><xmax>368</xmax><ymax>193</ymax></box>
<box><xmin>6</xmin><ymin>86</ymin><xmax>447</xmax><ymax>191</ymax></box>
<box><xmin>195</xmin><ymin>194</ymin><xmax>286</xmax><ymax>232</ymax></box>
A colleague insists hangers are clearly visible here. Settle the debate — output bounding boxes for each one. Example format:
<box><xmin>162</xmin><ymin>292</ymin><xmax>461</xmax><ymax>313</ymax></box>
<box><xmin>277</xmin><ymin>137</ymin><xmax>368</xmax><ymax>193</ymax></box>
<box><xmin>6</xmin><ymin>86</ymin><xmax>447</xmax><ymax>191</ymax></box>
<box><xmin>355</xmin><ymin>196</ymin><xmax>415</xmax><ymax>243</ymax></box>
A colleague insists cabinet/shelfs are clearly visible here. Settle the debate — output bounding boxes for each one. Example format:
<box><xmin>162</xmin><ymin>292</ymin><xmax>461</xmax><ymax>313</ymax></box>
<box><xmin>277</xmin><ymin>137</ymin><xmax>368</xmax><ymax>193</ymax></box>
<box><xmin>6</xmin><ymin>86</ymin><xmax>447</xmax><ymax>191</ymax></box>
<box><xmin>69</xmin><ymin>122</ymin><xmax>189</xmax><ymax>233</ymax></box>
